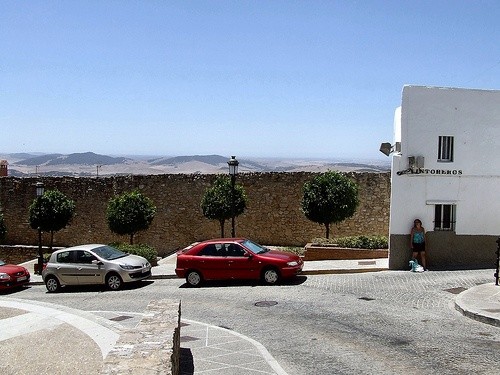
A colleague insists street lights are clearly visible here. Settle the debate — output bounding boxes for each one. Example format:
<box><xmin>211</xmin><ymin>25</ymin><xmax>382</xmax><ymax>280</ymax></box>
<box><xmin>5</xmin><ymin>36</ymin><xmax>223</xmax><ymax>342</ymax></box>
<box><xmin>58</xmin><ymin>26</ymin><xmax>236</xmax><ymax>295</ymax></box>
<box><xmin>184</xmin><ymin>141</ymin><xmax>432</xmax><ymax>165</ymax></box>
<box><xmin>33</xmin><ymin>182</ymin><xmax>46</xmax><ymax>274</ymax></box>
<box><xmin>227</xmin><ymin>155</ymin><xmax>239</xmax><ymax>237</ymax></box>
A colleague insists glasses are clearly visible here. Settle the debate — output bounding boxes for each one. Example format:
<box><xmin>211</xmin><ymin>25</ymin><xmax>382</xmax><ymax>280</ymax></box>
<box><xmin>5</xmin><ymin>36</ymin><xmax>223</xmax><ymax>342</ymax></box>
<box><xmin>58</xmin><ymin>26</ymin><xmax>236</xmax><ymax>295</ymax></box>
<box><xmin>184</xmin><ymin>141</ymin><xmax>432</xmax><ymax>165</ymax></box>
<box><xmin>415</xmin><ymin>222</ymin><xmax>420</xmax><ymax>223</ymax></box>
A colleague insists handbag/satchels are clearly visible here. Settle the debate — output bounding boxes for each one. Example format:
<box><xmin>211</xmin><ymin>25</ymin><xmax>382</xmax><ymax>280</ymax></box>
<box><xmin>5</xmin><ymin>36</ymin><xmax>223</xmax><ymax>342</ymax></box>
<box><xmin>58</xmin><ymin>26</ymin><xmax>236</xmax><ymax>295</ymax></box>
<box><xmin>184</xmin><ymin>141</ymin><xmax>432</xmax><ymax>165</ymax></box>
<box><xmin>408</xmin><ymin>259</ymin><xmax>424</xmax><ymax>273</ymax></box>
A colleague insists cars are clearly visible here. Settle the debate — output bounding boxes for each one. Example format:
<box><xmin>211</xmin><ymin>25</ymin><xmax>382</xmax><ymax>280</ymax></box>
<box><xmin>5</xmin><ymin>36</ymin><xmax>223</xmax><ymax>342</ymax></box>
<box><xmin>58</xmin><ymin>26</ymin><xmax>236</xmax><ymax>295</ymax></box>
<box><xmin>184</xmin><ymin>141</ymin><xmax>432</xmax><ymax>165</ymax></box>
<box><xmin>0</xmin><ymin>259</ymin><xmax>30</xmax><ymax>292</ymax></box>
<box><xmin>175</xmin><ymin>237</ymin><xmax>304</xmax><ymax>286</ymax></box>
<box><xmin>42</xmin><ymin>244</ymin><xmax>153</xmax><ymax>292</ymax></box>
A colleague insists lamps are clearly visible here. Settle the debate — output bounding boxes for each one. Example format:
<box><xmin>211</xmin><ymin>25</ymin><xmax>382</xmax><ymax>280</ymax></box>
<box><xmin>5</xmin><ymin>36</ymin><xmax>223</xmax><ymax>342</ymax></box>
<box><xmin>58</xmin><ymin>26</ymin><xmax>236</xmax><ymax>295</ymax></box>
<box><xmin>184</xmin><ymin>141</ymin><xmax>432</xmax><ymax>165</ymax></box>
<box><xmin>379</xmin><ymin>142</ymin><xmax>391</xmax><ymax>156</ymax></box>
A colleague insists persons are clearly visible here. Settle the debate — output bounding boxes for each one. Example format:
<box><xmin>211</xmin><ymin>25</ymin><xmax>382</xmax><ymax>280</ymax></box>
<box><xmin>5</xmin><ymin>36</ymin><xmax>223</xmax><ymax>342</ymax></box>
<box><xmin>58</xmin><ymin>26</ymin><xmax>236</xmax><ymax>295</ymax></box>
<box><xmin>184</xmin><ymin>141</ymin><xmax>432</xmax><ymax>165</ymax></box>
<box><xmin>410</xmin><ymin>218</ymin><xmax>428</xmax><ymax>271</ymax></box>
